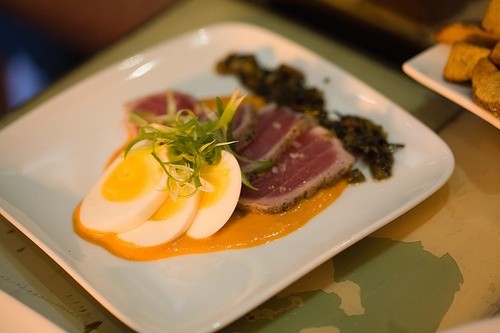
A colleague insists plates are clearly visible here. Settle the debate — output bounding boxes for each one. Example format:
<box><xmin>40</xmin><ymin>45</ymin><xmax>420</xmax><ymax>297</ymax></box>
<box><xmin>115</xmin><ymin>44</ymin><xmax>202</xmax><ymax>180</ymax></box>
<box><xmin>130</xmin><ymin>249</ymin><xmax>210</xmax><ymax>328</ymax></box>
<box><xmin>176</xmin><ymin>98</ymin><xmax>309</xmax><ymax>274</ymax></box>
<box><xmin>2</xmin><ymin>21</ymin><xmax>456</xmax><ymax>333</ymax></box>
<box><xmin>401</xmin><ymin>39</ymin><xmax>500</xmax><ymax>130</ymax></box>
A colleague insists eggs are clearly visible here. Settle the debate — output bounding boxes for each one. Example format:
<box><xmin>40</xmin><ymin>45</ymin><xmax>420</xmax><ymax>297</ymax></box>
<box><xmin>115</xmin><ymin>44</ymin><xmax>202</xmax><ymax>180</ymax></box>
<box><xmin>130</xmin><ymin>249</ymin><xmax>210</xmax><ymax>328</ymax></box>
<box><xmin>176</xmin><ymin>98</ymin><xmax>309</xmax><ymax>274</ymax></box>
<box><xmin>80</xmin><ymin>140</ymin><xmax>241</xmax><ymax>250</ymax></box>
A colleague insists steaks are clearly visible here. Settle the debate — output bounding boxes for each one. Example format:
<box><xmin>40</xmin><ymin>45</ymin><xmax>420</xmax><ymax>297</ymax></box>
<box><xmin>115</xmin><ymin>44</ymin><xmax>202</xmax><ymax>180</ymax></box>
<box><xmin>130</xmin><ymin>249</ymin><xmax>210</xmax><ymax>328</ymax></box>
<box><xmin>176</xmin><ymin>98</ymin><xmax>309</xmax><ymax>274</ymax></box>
<box><xmin>125</xmin><ymin>88</ymin><xmax>354</xmax><ymax>214</ymax></box>
